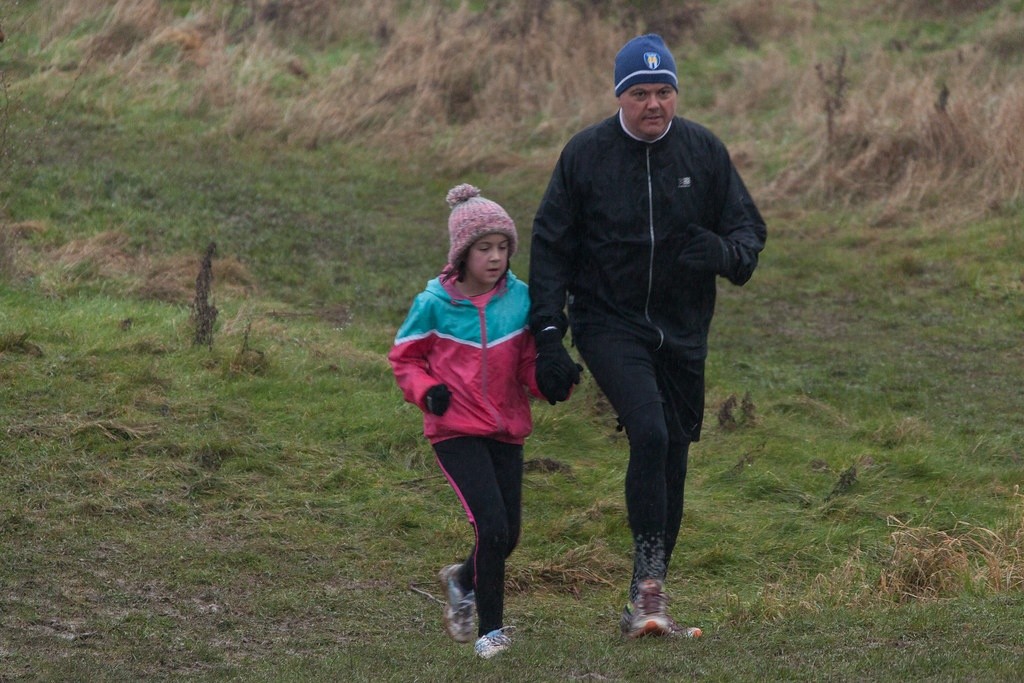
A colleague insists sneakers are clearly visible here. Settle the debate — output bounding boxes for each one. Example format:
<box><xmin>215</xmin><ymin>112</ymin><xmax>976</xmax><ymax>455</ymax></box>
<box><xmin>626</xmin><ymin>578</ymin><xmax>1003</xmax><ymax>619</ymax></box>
<box><xmin>628</xmin><ymin>578</ymin><xmax>674</xmax><ymax>638</ymax></box>
<box><xmin>438</xmin><ymin>564</ymin><xmax>476</xmax><ymax>643</ymax></box>
<box><xmin>473</xmin><ymin>625</ymin><xmax>516</xmax><ymax>662</ymax></box>
<box><xmin>620</xmin><ymin>603</ymin><xmax>703</xmax><ymax>640</ymax></box>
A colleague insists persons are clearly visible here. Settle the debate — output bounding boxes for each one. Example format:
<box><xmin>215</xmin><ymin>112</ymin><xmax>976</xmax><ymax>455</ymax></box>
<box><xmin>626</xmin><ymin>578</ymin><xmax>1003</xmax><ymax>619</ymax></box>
<box><xmin>527</xmin><ymin>34</ymin><xmax>767</xmax><ymax>641</ymax></box>
<box><xmin>388</xmin><ymin>184</ymin><xmax>575</xmax><ymax>661</ymax></box>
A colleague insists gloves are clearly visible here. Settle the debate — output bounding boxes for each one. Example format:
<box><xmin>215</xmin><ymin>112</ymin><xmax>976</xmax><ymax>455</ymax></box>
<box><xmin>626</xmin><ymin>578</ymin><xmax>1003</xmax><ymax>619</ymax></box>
<box><xmin>534</xmin><ymin>325</ymin><xmax>578</xmax><ymax>403</ymax></box>
<box><xmin>681</xmin><ymin>223</ymin><xmax>735</xmax><ymax>278</ymax></box>
<box><xmin>425</xmin><ymin>383</ymin><xmax>451</xmax><ymax>416</ymax></box>
<box><xmin>544</xmin><ymin>363</ymin><xmax>574</xmax><ymax>390</ymax></box>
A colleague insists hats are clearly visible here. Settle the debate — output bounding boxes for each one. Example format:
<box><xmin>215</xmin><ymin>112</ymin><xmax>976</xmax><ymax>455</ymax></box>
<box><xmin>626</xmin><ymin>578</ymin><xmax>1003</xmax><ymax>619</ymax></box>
<box><xmin>613</xmin><ymin>33</ymin><xmax>679</xmax><ymax>97</ymax></box>
<box><xmin>446</xmin><ymin>181</ymin><xmax>519</xmax><ymax>269</ymax></box>
